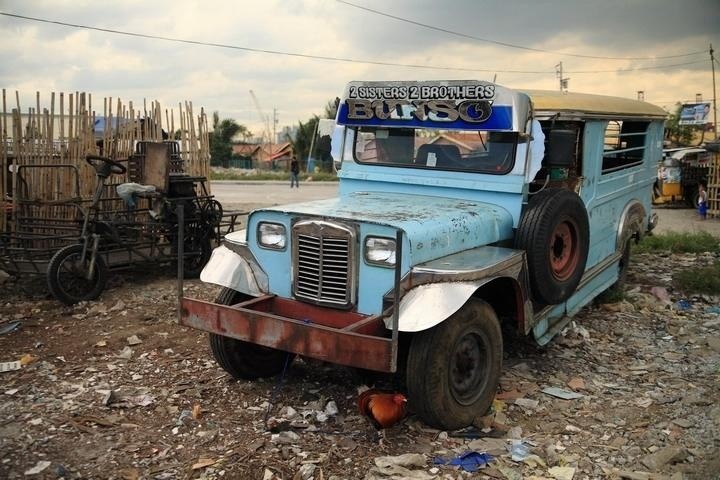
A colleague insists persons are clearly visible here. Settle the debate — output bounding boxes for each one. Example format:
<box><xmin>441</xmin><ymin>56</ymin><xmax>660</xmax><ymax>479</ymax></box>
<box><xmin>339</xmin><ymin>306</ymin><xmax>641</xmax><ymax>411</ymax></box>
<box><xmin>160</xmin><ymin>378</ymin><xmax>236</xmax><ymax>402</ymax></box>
<box><xmin>290</xmin><ymin>156</ymin><xmax>299</xmax><ymax>188</ymax></box>
<box><xmin>695</xmin><ymin>182</ymin><xmax>708</xmax><ymax>221</ymax></box>
<box><xmin>694</xmin><ymin>103</ymin><xmax>709</xmax><ymax>124</ymax></box>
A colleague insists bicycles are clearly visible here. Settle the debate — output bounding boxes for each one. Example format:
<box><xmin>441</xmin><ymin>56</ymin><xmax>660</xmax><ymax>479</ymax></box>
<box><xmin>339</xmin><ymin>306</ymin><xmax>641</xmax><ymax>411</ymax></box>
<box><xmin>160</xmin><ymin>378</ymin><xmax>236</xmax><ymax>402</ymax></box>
<box><xmin>45</xmin><ymin>152</ymin><xmax>215</xmax><ymax>307</ymax></box>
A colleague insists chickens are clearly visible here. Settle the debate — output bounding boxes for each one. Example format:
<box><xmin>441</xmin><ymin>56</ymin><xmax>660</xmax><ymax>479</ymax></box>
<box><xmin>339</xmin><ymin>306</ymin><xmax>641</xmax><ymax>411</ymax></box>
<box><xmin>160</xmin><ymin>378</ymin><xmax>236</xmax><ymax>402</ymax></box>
<box><xmin>355</xmin><ymin>382</ymin><xmax>408</xmax><ymax>450</ymax></box>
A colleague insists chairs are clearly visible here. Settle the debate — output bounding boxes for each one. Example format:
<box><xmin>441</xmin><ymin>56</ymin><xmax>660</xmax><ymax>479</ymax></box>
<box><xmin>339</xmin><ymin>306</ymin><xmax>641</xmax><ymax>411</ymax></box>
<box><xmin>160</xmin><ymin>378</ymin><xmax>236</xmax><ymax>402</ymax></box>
<box><xmin>415</xmin><ymin>144</ymin><xmax>462</xmax><ymax>167</ymax></box>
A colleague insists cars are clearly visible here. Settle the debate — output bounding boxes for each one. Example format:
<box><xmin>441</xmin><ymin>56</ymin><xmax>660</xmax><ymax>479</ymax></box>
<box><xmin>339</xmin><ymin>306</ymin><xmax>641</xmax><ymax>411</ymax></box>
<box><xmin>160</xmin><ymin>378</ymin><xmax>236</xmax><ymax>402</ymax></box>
<box><xmin>652</xmin><ymin>147</ymin><xmax>720</xmax><ymax>209</ymax></box>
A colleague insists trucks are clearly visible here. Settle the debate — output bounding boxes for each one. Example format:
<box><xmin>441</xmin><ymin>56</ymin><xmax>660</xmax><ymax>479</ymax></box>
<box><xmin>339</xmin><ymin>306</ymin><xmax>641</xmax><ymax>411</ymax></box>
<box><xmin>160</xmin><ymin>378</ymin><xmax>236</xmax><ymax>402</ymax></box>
<box><xmin>177</xmin><ymin>79</ymin><xmax>674</xmax><ymax>432</ymax></box>
